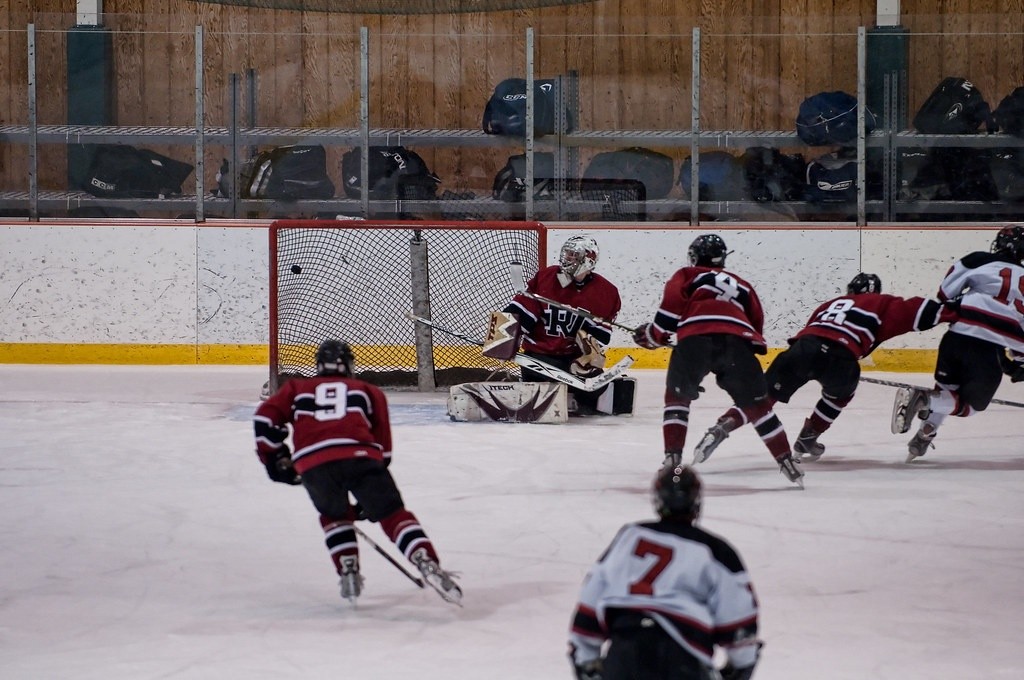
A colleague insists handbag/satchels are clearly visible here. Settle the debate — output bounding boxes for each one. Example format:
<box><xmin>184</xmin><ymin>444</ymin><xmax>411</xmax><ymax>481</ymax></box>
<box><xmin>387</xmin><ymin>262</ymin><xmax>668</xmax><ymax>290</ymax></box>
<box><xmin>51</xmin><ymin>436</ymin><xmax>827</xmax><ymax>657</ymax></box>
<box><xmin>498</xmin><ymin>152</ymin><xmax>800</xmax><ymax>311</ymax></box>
<box><xmin>83</xmin><ymin>76</ymin><xmax>1024</xmax><ymax>206</ymax></box>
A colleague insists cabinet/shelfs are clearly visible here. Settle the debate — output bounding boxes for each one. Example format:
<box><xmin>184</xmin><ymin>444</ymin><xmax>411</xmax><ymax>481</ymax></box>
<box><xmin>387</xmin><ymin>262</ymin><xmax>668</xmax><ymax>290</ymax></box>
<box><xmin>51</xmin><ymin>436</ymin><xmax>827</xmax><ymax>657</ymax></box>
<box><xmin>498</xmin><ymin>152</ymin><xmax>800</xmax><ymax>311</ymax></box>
<box><xmin>0</xmin><ymin>70</ymin><xmax>1024</xmax><ymax>221</ymax></box>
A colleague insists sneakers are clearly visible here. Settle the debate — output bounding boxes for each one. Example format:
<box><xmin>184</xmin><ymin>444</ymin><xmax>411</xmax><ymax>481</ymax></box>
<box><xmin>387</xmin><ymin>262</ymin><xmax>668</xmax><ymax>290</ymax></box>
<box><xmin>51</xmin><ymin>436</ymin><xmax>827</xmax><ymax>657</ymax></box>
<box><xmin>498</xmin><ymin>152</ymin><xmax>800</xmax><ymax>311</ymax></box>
<box><xmin>691</xmin><ymin>424</ymin><xmax>729</xmax><ymax>466</ymax></box>
<box><xmin>792</xmin><ymin>418</ymin><xmax>825</xmax><ymax>462</ymax></box>
<box><xmin>891</xmin><ymin>387</ymin><xmax>929</xmax><ymax>434</ymax></box>
<box><xmin>418</xmin><ymin>556</ymin><xmax>464</xmax><ymax>608</ymax></box>
<box><xmin>905</xmin><ymin>429</ymin><xmax>937</xmax><ymax>462</ymax></box>
<box><xmin>779</xmin><ymin>459</ymin><xmax>805</xmax><ymax>490</ymax></box>
<box><xmin>340</xmin><ymin>555</ymin><xmax>360</xmax><ymax>603</ymax></box>
<box><xmin>662</xmin><ymin>451</ymin><xmax>682</xmax><ymax>467</ymax></box>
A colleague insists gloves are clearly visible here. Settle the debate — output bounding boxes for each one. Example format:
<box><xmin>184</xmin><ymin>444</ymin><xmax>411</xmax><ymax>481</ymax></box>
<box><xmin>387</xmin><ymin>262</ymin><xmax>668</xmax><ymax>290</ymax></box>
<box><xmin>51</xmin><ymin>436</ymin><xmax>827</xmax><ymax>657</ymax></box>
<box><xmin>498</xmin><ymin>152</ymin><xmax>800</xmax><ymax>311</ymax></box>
<box><xmin>632</xmin><ymin>323</ymin><xmax>662</xmax><ymax>349</ymax></box>
<box><xmin>266</xmin><ymin>451</ymin><xmax>301</xmax><ymax>485</ymax></box>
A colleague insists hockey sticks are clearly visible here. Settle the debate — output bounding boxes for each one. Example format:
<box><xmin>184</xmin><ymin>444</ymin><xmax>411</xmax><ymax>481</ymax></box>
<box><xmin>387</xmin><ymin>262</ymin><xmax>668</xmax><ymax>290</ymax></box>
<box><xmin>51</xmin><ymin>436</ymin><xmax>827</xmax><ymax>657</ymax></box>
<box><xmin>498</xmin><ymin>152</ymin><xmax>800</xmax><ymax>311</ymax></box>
<box><xmin>404</xmin><ymin>312</ymin><xmax>636</xmax><ymax>393</ymax></box>
<box><xmin>511</xmin><ymin>261</ymin><xmax>676</xmax><ymax>349</ymax></box>
<box><xmin>281</xmin><ymin>465</ymin><xmax>431</xmax><ymax>588</ymax></box>
<box><xmin>859</xmin><ymin>376</ymin><xmax>1024</xmax><ymax>408</ymax></box>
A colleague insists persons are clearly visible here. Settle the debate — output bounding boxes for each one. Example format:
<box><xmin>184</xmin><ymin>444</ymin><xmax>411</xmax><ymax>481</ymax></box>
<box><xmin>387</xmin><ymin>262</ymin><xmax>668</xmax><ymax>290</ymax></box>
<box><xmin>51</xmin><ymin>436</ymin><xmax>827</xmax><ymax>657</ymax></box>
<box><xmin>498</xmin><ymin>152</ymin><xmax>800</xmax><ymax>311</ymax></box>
<box><xmin>891</xmin><ymin>224</ymin><xmax>1024</xmax><ymax>467</ymax></box>
<box><xmin>566</xmin><ymin>465</ymin><xmax>765</xmax><ymax>680</ymax></box>
<box><xmin>251</xmin><ymin>341</ymin><xmax>465</xmax><ymax>606</ymax></box>
<box><xmin>501</xmin><ymin>234</ymin><xmax>622</xmax><ymax>417</ymax></box>
<box><xmin>632</xmin><ymin>235</ymin><xmax>806</xmax><ymax>496</ymax></box>
<box><xmin>692</xmin><ymin>272</ymin><xmax>959</xmax><ymax>465</ymax></box>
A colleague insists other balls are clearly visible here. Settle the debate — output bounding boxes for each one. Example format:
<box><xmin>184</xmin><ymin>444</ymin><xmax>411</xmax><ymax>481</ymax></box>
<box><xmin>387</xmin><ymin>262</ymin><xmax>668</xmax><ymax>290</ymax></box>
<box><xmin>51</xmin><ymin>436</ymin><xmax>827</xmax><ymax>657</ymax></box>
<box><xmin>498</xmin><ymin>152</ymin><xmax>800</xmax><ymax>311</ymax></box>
<box><xmin>291</xmin><ymin>265</ymin><xmax>302</xmax><ymax>274</ymax></box>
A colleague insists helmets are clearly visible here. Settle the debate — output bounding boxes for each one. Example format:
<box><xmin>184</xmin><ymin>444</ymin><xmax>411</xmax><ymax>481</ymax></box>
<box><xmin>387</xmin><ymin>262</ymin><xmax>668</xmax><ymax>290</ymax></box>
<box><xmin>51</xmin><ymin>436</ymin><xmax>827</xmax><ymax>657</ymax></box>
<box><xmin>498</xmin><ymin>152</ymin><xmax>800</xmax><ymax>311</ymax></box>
<box><xmin>996</xmin><ymin>226</ymin><xmax>1024</xmax><ymax>260</ymax></box>
<box><xmin>848</xmin><ymin>273</ymin><xmax>881</xmax><ymax>294</ymax></box>
<box><xmin>315</xmin><ymin>340</ymin><xmax>355</xmax><ymax>375</ymax></box>
<box><xmin>562</xmin><ymin>233</ymin><xmax>599</xmax><ymax>275</ymax></box>
<box><xmin>657</xmin><ymin>465</ymin><xmax>701</xmax><ymax>518</ymax></box>
<box><xmin>688</xmin><ymin>234</ymin><xmax>727</xmax><ymax>268</ymax></box>
